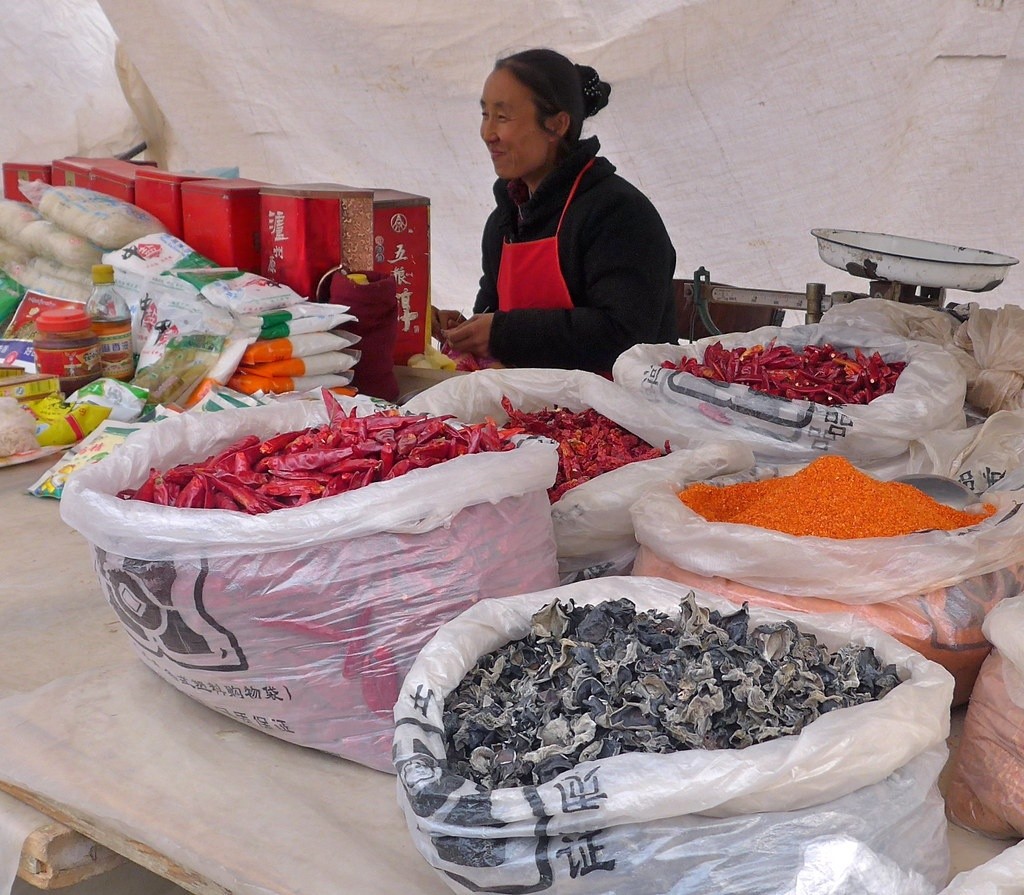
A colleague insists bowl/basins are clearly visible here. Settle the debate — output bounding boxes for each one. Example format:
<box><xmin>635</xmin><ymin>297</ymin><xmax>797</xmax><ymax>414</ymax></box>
<box><xmin>810</xmin><ymin>227</ymin><xmax>1020</xmax><ymax>292</ymax></box>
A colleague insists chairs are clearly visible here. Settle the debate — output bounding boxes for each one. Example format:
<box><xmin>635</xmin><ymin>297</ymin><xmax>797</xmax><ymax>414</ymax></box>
<box><xmin>671</xmin><ymin>276</ymin><xmax>788</xmax><ymax>346</ymax></box>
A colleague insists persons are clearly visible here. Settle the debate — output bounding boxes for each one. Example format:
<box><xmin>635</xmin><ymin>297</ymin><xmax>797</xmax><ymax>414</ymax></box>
<box><xmin>431</xmin><ymin>48</ymin><xmax>677</xmax><ymax>382</ymax></box>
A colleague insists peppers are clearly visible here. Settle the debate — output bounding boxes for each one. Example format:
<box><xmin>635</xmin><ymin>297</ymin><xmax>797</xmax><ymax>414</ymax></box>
<box><xmin>107</xmin><ymin>388</ymin><xmax>564</xmax><ymax>775</ymax></box>
<box><xmin>661</xmin><ymin>337</ymin><xmax>908</xmax><ymax>406</ymax></box>
<box><xmin>495</xmin><ymin>392</ymin><xmax>672</xmax><ymax>503</ymax></box>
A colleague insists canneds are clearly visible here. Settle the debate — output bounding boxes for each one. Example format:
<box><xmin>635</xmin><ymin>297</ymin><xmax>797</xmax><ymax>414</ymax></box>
<box><xmin>32</xmin><ymin>309</ymin><xmax>102</xmax><ymax>396</ymax></box>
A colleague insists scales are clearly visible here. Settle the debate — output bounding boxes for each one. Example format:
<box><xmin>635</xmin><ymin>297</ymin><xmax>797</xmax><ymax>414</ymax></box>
<box><xmin>683</xmin><ymin>228</ymin><xmax>1020</xmax><ymax>344</ymax></box>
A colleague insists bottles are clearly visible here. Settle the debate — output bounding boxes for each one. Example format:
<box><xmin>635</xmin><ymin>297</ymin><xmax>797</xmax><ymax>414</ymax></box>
<box><xmin>84</xmin><ymin>264</ymin><xmax>135</xmax><ymax>382</ymax></box>
<box><xmin>32</xmin><ymin>308</ymin><xmax>102</xmax><ymax>398</ymax></box>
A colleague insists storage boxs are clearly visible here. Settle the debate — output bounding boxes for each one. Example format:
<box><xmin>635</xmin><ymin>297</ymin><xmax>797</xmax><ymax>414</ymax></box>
<box><xmin>3</xmin><ymin>156</ymin><xmax>433</xmax><ymax>398</ymax></box>
